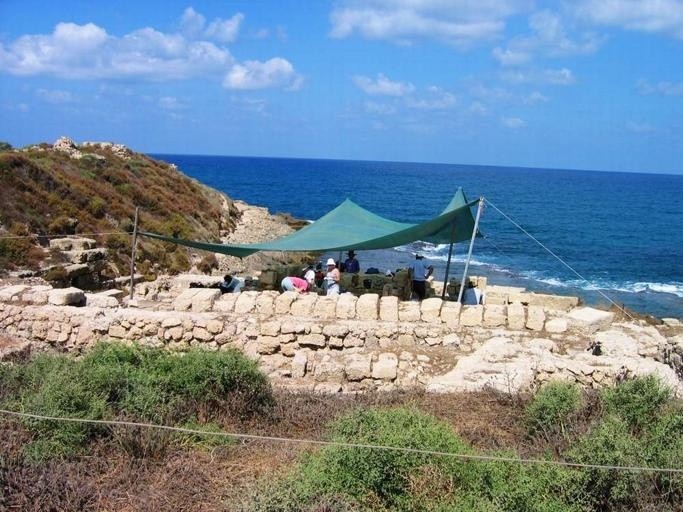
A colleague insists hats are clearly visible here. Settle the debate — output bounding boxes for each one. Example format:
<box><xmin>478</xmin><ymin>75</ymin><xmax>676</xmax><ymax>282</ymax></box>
<box><xmin>345</xmin><ymin>250</ymin><xmax>358</xmax><ymax>256</ymax></box>
<box><xmin>416</xmin><ymin>253</ymin><xmax>425</xmax><ymax>258</ymax></box>
<box><xmin>325</xmin><ymin>258</ymin><xmax>336</xmax><ymax>266</ymax></box>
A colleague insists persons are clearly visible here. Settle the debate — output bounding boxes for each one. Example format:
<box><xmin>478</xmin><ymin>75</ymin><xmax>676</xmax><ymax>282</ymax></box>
<box><xmin>217</xmin><ymin>274</ymin><xmax>241</xmax><ymax>293</ymax></box>
<box><xmin>281</xmin><ymin>249</ymin><xmax>360</xmax><ymax>297</ymax></box>
<box><xmin>460</xmin><ymin>281</ymin><xmax>477</xmax><ymax>305</ymax></box>
<box><xmin>408</xmin><ymin>253</ymin><xmax>433</xmax><ymax>301</ymax></box>
